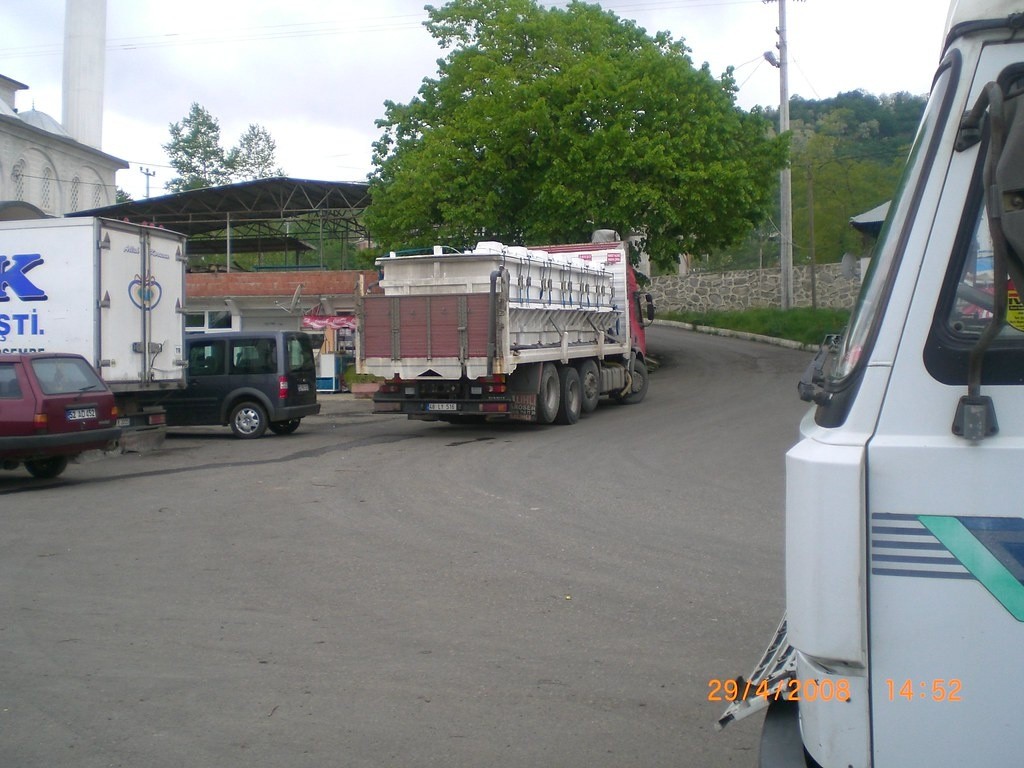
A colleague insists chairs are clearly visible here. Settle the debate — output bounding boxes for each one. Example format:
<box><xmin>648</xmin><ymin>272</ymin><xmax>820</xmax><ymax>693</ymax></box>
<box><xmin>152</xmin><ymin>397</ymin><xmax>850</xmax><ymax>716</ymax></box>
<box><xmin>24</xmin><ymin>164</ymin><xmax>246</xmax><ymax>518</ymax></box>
<box><xmin>191</xmin><ymin>354</ymin><xmax>217</xmax><ymax>375</ymax></box>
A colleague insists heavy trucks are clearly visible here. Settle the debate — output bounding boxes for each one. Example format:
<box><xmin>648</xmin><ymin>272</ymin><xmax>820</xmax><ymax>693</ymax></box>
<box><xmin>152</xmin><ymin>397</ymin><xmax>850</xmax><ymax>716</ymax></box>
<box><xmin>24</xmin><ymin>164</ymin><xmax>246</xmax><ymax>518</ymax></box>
<box><xmin>356</xmin><ymin>240</ymin><xmax>656</xmax><ymax>427</ymax></box>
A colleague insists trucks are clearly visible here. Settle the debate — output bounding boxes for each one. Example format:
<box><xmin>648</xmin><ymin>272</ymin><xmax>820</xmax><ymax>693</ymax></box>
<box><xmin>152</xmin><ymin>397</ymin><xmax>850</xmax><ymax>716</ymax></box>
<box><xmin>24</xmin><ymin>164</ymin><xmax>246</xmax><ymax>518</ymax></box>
<box><xmin>713</xmin><ymin>0</ymin><xmax>1024</xmax><ymax>768</ymax></box>
<box><xmin>0</xmin><ymin>216</ymin><xmax>190</xmax><ymax>435</ymax></box>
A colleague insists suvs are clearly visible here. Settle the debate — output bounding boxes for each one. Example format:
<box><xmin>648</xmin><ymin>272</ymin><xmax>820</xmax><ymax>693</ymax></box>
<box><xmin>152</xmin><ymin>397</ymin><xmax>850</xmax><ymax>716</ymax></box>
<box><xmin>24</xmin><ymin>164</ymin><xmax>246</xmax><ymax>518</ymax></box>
<box><xmin>0</xmin><ymin>352</ymin><xmax>123</xmax><ymax>479</ymax></box>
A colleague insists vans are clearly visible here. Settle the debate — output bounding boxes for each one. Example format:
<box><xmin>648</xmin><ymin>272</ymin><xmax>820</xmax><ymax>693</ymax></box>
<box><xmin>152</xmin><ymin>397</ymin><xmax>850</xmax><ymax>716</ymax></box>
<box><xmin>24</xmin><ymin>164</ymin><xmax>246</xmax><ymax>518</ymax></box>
<box><xmin>136</xmin><ymin>329</ymin><xmax>320</xmax><ymax>440</ymax></box>
